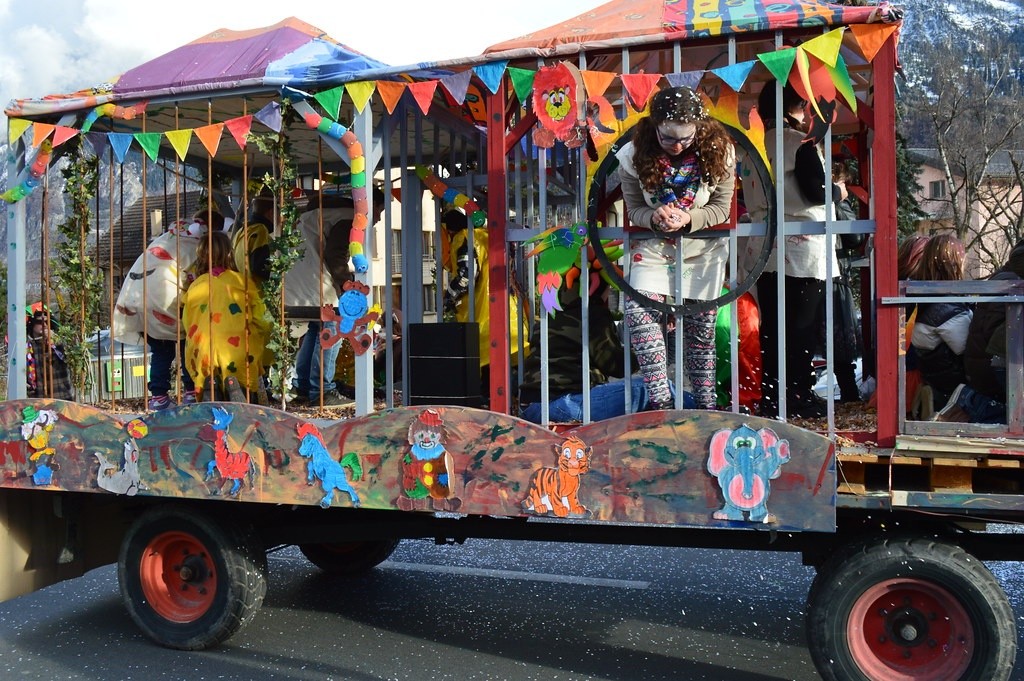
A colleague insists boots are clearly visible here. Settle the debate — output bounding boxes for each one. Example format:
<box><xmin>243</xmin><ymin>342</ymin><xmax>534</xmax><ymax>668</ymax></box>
<box><xmin>834</xmin><ymin>362</ymin><xmax>877</xmax><ymax>404</ymax></box>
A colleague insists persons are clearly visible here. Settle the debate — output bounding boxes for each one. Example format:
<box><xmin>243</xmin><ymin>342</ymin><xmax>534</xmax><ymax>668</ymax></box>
<box><xmin>20</xmin><ymin>181</ymin><xmax>525</xmax><ymax>415</ymax></box>
<box><xmin>742</xmin><ymin>77</ymin><xmax>1024</xmax><ymax>421</ymax></box>
<box><xmin>613</xmin><ymin>88</ymin><xmax>739</xmax><ymax>414</ymax></box>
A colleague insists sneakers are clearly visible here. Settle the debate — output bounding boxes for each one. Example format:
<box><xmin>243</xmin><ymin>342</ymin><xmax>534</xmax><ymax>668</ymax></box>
<box><xmin>183</xmin><ymin>391</ymin><xmax>196</xmax><ymax>405</ymax></box>
<box><xmin>309</xmin><ymin>387</ymin><xmax>355</xmax><ymax>410</ymax></box>
<box><xmin>289</xmin><ymin>385</ymin><xmax>320</xmax><ymax>399</ymax></box>
<box><xmin>148</xmin><ymin>394</ymin><xmax>178</xmax><ymax>415</ymax></box>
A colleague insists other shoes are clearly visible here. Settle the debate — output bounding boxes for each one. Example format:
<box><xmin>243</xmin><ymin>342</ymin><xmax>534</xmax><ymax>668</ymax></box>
<box><xmin>724</xmin><ymin>404</ymin><xmax>751</xmax><ymax>416</ymax></box>
<box><xmin>930</xmin><ymin>383</ymin><xmax>989</xmax><ymax>422</ymax></box>
<box><xmin>223</xmin><ymin>376</ymin><xmax>247</xmax><ymax>402</ymax></box>
<box><xmin>787</xmin><ymin>388</ymin><xmax>826</xmax><ymax>419</ymax></box>
<box><xmin>201</xmin><ymin>376</ymin><xmax>225</xmax><ymax>402</ymax></box>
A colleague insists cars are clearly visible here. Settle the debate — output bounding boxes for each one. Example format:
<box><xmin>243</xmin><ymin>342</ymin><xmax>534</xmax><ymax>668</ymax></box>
<box><xmin>83</xmin><ymin>327</ymin><xmax>152</xmax><ymax>359</ymax></box>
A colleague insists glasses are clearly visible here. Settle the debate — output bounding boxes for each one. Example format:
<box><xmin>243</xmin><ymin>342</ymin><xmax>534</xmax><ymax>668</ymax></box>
<box><xmin>657</xmin><ymin>125</ymin><xmax>695</xmax><ymax>147</ymax></box>
<box><xmin>34</xmin><ymin>319</ymin><xmax>48</xmax><ymax>325</ymax></box>
